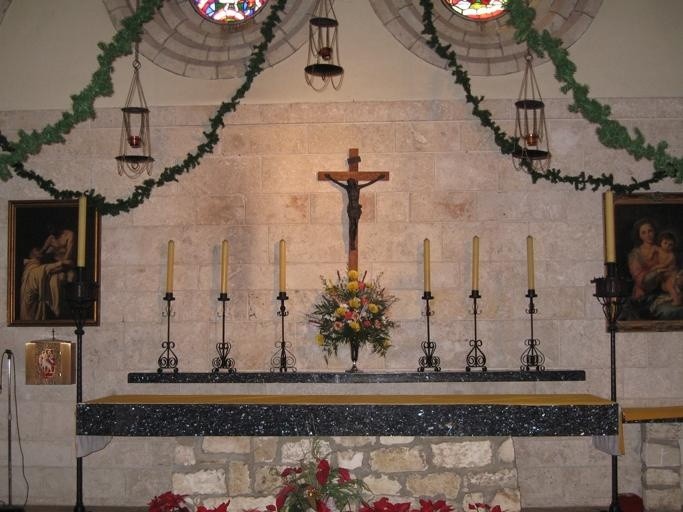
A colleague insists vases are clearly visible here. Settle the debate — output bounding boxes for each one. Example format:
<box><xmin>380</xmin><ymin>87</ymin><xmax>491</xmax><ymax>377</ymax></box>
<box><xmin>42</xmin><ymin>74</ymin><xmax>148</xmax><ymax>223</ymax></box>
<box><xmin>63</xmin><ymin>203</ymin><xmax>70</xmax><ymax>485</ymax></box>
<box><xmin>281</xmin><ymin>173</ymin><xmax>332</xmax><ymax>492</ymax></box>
<box><xmin>345</xmin><ymin>337</ymin><xmax>363</xmax><ymax>375</ymax></box>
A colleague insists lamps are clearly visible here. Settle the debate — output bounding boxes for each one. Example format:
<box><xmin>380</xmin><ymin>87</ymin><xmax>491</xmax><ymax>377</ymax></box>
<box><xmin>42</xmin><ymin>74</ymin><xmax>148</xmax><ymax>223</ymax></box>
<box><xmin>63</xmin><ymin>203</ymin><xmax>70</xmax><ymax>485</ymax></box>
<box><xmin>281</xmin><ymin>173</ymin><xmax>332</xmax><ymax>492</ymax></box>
<box><xmin>114</xmin><ymin>24</ymin><xmax>156</xmax><ymax>178</ymax></box>
<box><xmin>511</xmin><ymin>44</ymin><xmax>553</xmax><ymax>178</ymax></box>
<box><xmin>304</xmin><ymin>0</ymin><xmax>343</xmax><ymax>93</ymax></box>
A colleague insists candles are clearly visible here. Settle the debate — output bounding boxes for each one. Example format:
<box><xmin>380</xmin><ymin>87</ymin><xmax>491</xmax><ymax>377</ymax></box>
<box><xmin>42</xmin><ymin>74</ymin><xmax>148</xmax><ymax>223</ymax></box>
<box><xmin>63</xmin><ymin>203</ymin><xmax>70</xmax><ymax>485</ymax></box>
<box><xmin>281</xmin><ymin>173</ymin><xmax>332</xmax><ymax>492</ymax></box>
<box><xmin>604</xmin><ymin>189</ymin><xmax>617</xmax><ymax>265</ymax></box>
<box><xmin>424</xmin><ymin>237</ymin><xmax>430</xmax><ymax>293</ymax></box>
<box><xmin>472</xmin><ymin>235</ymin><xmax>480</xmax><ymax>291</ymax></box>
<box><xmin>278</xmin><ymin>238</ymin><xmax>286</xmax><ymax>294</ymax></box>
<box><xmin>166</xmin><ymin>239</ymin><xmax>175</xmax><ymax>295</ymax></box>
<box><xmin>527</xmin><ymin>236</ymin><xmax>534</xmax><ymax>291</ymax></box>
<box><xmin>77</xmin><ymin>192</ymin><xmax>88</xmax><ymax>266</ymax></box>
<box><xmin>221</xmin><ymin>239</ymin><xmax>227</xmax><ymax>294</ymax></box>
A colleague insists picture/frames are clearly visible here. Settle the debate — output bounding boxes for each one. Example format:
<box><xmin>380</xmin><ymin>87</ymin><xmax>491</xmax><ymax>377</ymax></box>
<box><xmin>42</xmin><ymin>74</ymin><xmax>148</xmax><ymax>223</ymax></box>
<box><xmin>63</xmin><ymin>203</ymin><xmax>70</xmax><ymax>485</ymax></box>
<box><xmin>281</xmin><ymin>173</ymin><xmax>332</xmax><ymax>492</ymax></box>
<box><xmin>7</xmin><ymin>198</ymin><xmax>99</xmax><ymax>326</ymax></box>
<box><xmin>603</xmin><ymin>193</ymin><xmax>683</xmax><ymax>333</ymax></box>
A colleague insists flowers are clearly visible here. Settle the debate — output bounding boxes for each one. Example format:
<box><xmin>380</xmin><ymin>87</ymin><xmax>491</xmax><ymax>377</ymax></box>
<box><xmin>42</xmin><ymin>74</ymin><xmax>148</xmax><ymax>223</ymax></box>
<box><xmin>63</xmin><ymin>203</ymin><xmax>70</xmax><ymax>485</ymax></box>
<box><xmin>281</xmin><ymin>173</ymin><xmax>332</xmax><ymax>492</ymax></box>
<box><xmin>265</xmin><ymin>412</ymin><xmax>375</xmax><ymax>512</ymax></box>
<box><xmin>304</xmin><ymin>265</ymin><xmax>401</xmax><ymax>363</ymax></box>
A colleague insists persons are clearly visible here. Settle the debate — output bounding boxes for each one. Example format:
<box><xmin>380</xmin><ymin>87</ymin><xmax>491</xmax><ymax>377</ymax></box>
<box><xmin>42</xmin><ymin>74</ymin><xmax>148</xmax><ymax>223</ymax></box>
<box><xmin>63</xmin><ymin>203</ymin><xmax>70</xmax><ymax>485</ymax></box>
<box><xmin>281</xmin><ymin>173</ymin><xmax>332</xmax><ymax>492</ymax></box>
<box><xmin>19</xmin><ymin>219</ymin><xmax>76</xmax><ymax>323</ymax></box>
<box><xmin>323</xmin><ymin>172</ymin><xmax>384</xmax><ymax>250</ymax></box>
<box><xmin>628</xmin><ymin>222</ymin><xmax>683</xmax><ymax>321</ymax></box>
